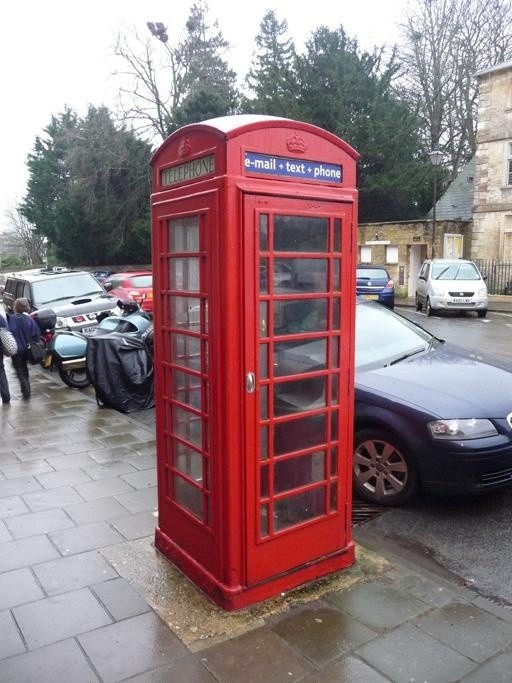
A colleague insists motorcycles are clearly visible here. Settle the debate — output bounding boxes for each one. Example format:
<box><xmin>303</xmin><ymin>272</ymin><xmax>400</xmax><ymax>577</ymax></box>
<box><xmin>29</xmin><ymin>293</ymin><xmax>150</xmax><ymax>389</ymax></box>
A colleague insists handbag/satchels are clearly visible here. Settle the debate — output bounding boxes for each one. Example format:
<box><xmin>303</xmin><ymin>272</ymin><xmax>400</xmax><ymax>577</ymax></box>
<box><xmin>0</xmin><ymin>328</ymin><xmax>18</xmax><ymax>356</ymax></box>
<box><xmin>24</xmin><ymin>342</ymin><xmax>44</xmax><ymax>365</ymax></box>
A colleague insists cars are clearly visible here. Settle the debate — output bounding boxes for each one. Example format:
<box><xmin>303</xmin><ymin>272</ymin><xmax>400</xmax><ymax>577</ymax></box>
<box><xmin>3</xmin><ymin>271</ymin><xmax>153</xmax><ymax>317</ymax></box>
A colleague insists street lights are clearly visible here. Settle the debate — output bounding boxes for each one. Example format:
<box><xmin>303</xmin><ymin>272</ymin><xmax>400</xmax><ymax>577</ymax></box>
<box><xmin>428</xmin><ymin>148</ymin><xmax>443</xmax><ymax>258</ymax></box>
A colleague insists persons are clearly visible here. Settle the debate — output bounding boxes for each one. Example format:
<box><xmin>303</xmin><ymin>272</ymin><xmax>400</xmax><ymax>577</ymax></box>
<box><xmin>0</xmin><ymin>312</ymin><xmax>12</xmax><ymax>405</ymax></box>
<box><xmin>8</xmin><ymin>297</ymin><xmax>43</xmax><ymax>403</ymax></box>
